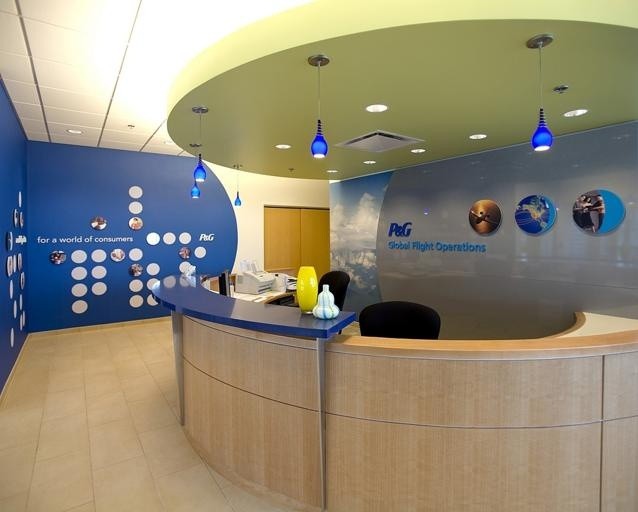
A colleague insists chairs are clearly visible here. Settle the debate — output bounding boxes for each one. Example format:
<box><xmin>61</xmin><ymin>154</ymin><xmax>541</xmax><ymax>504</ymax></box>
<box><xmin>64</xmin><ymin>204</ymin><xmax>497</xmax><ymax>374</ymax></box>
<box><xmin>313</xmin><ymin>270</ymin><xmax>442</xmax><ymax>341</ymax></box>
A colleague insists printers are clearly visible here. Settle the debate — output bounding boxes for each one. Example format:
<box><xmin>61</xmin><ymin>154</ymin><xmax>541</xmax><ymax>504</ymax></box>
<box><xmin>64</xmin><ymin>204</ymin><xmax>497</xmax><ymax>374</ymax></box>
<box><xmin>234</xmin><ymin>270</ymin><xmax>277</xmax><ymax>295</ymax></box>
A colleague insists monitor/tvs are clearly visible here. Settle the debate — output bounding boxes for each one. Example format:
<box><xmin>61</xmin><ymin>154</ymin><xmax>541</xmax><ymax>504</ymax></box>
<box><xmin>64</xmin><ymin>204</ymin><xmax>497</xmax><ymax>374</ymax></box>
<box><xmin>218</xmin><ymin>271</ymin><xmax>231</xmax><ymax>297</ymax></box>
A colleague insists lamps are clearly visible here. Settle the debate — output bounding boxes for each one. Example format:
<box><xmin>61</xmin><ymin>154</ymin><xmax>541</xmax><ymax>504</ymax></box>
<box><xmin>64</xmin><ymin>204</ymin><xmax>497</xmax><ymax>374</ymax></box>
<box><xmin>234</xmin><ymin>165</ymin><xmax>242</xmax><ymax>206</ymax></box>
<box><xmin>525</xmin><ymin>33</ymin><xmax>555</xmax><ymax>153</ymax></box>
<box><xmin>179</xmin><ymin>106</ymin><xmax>210</xmax><ymax>199</ymax></box>
<box><xmin>308</xmin><ymin>53</ymin><xmax>331</xmax><ymax>159</ymax></box>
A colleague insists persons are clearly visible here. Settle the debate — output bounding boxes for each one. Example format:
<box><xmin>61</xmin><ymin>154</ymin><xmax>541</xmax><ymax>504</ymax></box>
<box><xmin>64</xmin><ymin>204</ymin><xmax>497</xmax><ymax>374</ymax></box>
<box><xmin>573</xmin><ymin>194</ymin><xmax>605</xmax><ymax>233</ymax></box>
<box><xmin>471</xmin><ymin>210</ymin><xmax>499</xmax><ymax>225</ymax></box>
